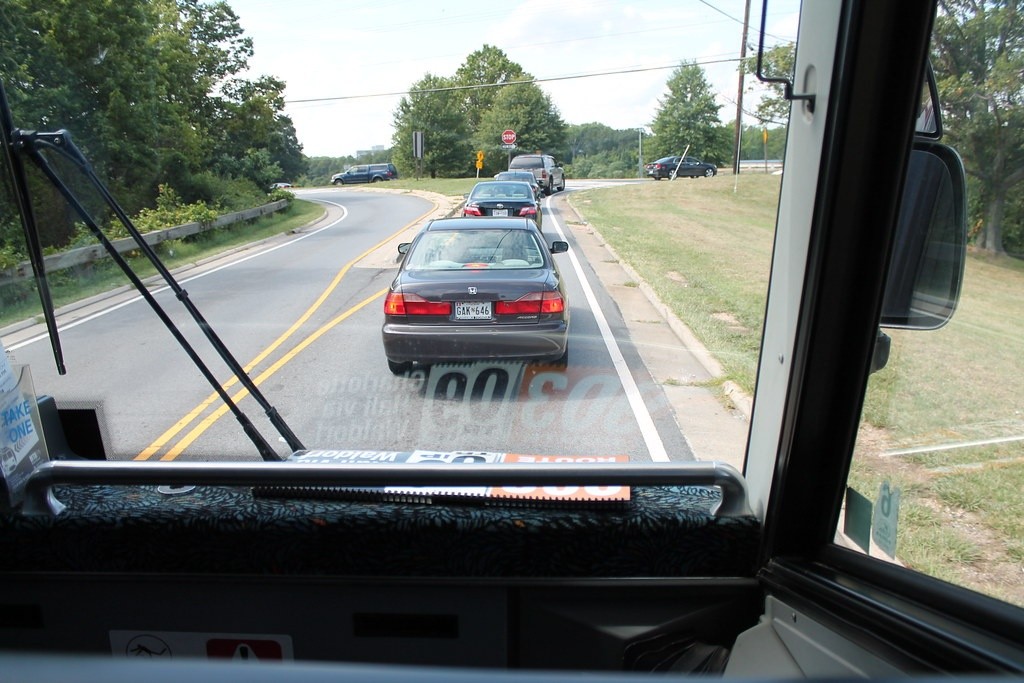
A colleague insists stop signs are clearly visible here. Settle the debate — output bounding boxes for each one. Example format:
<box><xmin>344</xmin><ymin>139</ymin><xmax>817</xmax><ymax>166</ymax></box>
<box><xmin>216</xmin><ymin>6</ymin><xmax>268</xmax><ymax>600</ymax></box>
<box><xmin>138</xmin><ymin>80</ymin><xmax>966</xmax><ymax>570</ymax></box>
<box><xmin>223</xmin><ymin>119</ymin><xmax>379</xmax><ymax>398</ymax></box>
<box><xmin>502</xmin><ymin>130</ymin><xmax>516</xmax><ymax>144</ymax></box>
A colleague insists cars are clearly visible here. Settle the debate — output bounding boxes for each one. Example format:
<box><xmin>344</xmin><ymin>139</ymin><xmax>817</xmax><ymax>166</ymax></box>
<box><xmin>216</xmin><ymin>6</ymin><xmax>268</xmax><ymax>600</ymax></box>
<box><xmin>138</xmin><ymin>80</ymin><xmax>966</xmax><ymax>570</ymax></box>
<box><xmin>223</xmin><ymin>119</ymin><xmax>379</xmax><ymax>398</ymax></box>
<box><xmin>494</xmin><ymin>171</ymin><xmax>544</xmax><ymax>201</ymax></box>
<box><xmin>645</xmin><ymin>155</ymin><xmax>718</xmax><ymax>181</ymax></box>
<box><xmin>461</xmin><ymin>180</ymin><xmax>545</xmax><ymax>233</ymax></box>
<box><xmin>380</xmin><ymin>214</ymin><xmax>571</xmax><ymax>382</ymax></box>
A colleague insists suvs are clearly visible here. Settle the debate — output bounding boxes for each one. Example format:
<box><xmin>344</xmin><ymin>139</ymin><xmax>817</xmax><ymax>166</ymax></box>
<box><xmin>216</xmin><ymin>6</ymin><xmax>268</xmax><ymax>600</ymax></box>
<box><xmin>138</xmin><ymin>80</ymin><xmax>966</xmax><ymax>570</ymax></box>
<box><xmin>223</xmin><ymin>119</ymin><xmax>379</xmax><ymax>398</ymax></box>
<box><xmin>331</xmin><ymin>162</ymin><xmax>397</xmax><ymax>186</ymax></box>
<box><xmin>508</xmin><ymin>152</ymin><xmax>565</xmax><ymax>196</ymax></box>
